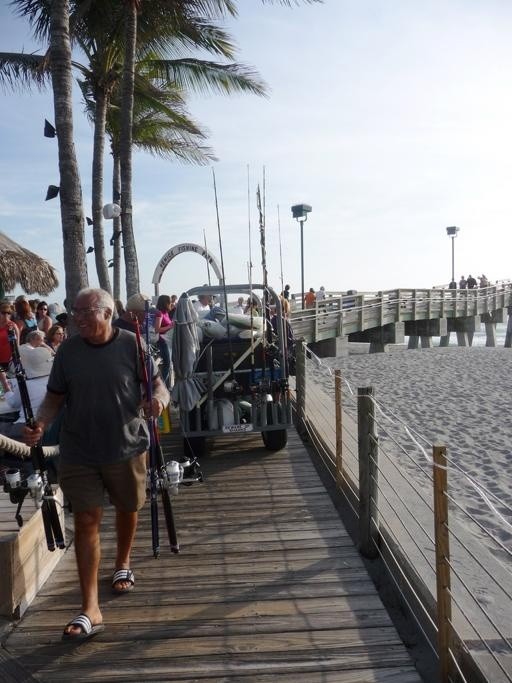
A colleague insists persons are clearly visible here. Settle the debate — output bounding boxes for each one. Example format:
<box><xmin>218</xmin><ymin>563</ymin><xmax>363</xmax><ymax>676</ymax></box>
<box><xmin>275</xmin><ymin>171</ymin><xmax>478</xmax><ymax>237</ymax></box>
<box><xmin>1</xmin><ymin>294</ymin><xmax>69</xmax><ymax>395</ymax></box>
<box><xmin>304</xmin><ymin>286</ymin><xmax>326</xmax><ymax>308</ymax></box>
<box><xmin>235</xmin><ymin>297</ymin><xmax>259</xmax><ymax>316</ymax></box>
<box><xmin>270</xmin><ymin>285</ymin><xmax>290</xmax><ymax>304</ymax></box>
<box><xmin>448</xmin><ymin>273</ymin><xmax>492</xmax><ymax>291</ymax></box>
<box><xmin>22</xmin><ymin>285</ymin><xmax>170</xmax><ymax>642</ymax></box>
<box><xmin>192</xmin><ymin>294</ymin><xmax>217</xmax><ymax>317</ymax></box>
<box><xmin>117</xmin><ymin>294</ymin><xmax>177</xmax><ymax>376</ymax></box>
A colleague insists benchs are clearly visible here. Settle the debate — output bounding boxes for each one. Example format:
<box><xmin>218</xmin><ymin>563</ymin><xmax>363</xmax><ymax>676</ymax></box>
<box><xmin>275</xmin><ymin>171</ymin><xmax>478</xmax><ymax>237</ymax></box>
<box><xmin>0</xmin><ymin>486</ymin><xmax>65</xmax><ymax>614</ymax></box>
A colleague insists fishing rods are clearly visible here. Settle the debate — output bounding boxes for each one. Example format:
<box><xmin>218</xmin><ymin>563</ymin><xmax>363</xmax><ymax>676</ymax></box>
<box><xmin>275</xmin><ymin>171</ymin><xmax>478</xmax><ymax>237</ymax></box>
<box><xmin>5</xmin><ymin>320</ymin><xmax>66</xmax><ymax>552</ymax></box>
<box><xmin>132</xmin><ymin>296</ymin><xmax>181</xmax><ymax>559</ymax></box>
<box><xmin>210</xmin><ymin>167</ymin><xmax>291</xmax><ymax>427</ymax></box>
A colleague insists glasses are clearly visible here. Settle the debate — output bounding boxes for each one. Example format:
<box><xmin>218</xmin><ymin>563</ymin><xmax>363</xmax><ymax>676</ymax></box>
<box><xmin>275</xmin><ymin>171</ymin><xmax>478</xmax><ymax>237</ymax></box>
<box><xmin>39</xmin><ymin>308</ymin><xmax>47</xmax><ymax>310</ymax></box>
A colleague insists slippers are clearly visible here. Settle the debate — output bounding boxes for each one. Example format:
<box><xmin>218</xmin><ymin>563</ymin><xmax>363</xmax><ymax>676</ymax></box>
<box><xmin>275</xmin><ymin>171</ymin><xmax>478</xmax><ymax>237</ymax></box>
<box><xmin>111</xmin><ymin>569</ymin><xmax>134</xmax><ymax>593</ymax></box>
<box><xmin>61</xmin><ymin>614</ymin><xmax>106</xmax><ymax>641</ymax></box>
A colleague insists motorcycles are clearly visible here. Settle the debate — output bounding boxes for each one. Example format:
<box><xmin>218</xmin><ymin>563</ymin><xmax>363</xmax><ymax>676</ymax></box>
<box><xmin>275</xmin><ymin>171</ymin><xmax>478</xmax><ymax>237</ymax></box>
<box><xmin>173</xmin><ymin>282</ymin><xmax>295</xmax><ymax>460</ymax></box>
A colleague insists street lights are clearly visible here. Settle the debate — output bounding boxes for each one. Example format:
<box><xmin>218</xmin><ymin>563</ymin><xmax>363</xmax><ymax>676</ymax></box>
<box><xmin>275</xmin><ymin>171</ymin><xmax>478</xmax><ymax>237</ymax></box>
<box><xmin>291</xmin><ymin>203</ymin><xmax>312</xmax><ymax>321</ymax></box>
<box><xmin>446</xmin><ymin>225</ymin><xmax>459</xmax><ymax>300</ymax></box>
